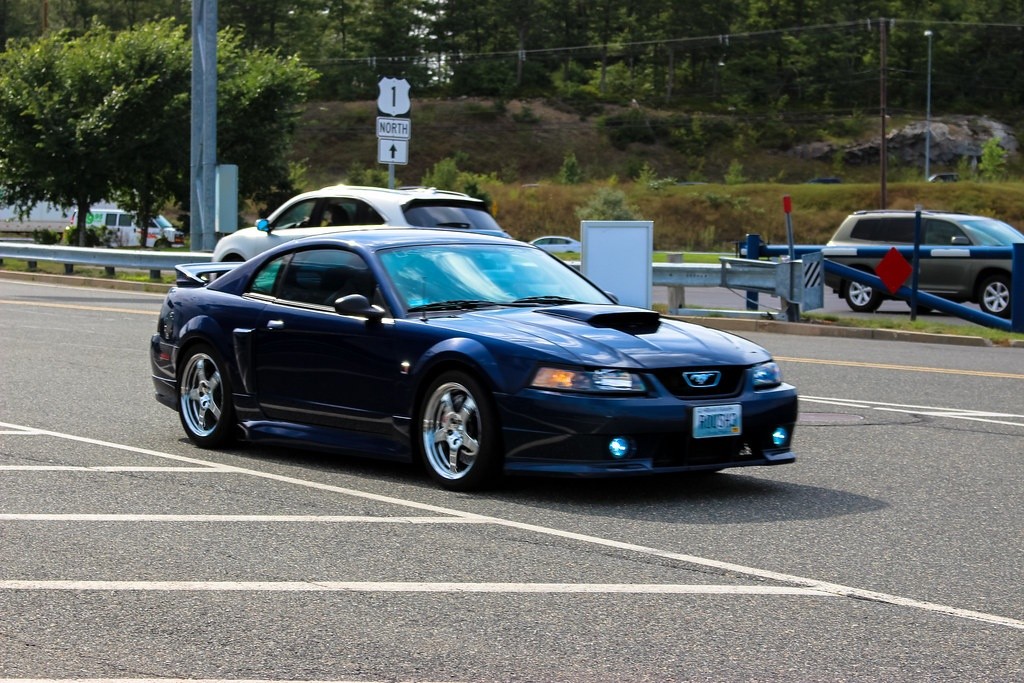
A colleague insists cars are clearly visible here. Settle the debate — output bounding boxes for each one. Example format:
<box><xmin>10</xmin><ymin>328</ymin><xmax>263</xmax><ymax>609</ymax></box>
<box><xmin>525</xmin><ymin>234</ymin><xmax>582</xmax><ymax>255</ymax></box>
<box><xmin>145</xmin><ymin>227</ymin><xmax>801</xmax><ymax>496</ymax></box>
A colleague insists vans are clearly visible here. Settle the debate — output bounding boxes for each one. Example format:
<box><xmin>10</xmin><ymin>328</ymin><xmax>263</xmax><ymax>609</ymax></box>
<box><xmin>70</xmin><ymin>206</ymin><xmax>183</xmax><ymax>251</ymax></box>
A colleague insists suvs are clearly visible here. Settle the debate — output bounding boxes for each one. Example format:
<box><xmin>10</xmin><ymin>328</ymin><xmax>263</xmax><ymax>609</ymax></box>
<box><xmin>208</xmin><ymin>181</ymin><xmax>503</xmax><ymax>299</ymax></box>
<box><xmin>823</xmin><ymin>211</ymin><xmax>1024</xmax><ymax>318</ymax></box>
